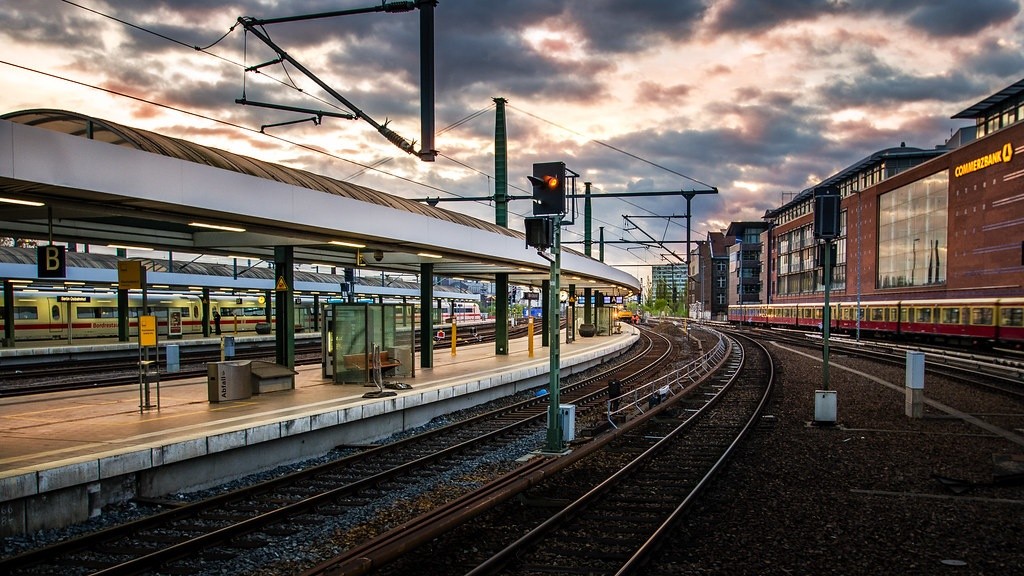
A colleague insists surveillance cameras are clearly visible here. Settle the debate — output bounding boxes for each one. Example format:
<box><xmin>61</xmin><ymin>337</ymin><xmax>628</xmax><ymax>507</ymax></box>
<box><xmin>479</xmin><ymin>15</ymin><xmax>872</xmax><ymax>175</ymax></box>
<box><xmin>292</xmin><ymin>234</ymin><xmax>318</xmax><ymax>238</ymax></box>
<box><xmin>374</xmin><ymin>251</ymin><xmax>383</xmax><ymax>262</ymax></box>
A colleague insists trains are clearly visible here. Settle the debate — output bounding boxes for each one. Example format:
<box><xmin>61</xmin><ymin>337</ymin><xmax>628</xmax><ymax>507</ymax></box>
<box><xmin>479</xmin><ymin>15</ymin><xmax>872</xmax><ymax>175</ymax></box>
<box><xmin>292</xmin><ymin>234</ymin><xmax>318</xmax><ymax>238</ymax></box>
<box><xmin>0</xmin><ymin>280</ymin><xmax>484</xmax><ymax>351</ymax></box>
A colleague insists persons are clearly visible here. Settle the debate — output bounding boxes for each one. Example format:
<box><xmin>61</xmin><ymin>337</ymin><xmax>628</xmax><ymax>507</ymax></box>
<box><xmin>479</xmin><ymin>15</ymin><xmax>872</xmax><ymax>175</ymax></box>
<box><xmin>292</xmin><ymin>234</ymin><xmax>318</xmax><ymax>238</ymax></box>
<box><xmin>214</xmin><ymin>312</ymin><xmax>221</xmax><ymax>335</ymax></box>
<box><xmin>631</xmin><ymin>309</ymin><xmax>643</xmax><ymax>325</ymax></box>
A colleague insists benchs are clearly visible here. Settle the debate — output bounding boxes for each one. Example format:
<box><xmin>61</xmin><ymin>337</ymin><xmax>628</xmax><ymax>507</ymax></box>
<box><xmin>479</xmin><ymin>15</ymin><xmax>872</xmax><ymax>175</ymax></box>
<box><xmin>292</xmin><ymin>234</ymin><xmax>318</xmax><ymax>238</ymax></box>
<box><xmin>295</xmin><ymin>324</ymin><xmax>304</xmax><ymax>333</ymax></box>
<box><xmin>343</xmin><ymin>351</ymin><xmax>402</xmax><ymax>385</ymax></box>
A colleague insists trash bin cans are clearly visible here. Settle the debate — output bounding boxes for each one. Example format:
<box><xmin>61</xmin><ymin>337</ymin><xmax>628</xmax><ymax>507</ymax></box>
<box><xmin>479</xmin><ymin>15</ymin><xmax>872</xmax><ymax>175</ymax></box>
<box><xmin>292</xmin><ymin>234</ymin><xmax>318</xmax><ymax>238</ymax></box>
<box><xmin>255</xmin><ymin>322</ymin><xmax>271</xmax><ymax>335</ymax></box>
<box><xmin>207</xmin><ymin>360</ymin><xmax>253</xmax><ymax>402</ymax></box>
<box><xmin>579</xmin><ymin>324</ymin><xmax>595</xmax><ymax>337</ymax></box>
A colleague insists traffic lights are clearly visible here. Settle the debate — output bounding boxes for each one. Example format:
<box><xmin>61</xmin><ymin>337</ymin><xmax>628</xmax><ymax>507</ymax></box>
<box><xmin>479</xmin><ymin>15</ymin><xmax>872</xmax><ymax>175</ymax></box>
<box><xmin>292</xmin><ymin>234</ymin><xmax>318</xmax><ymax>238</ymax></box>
<box><xmin>532</xmin><ymin>161</ymin><xmax>566</xmax><ymax>215</ymax></box>
